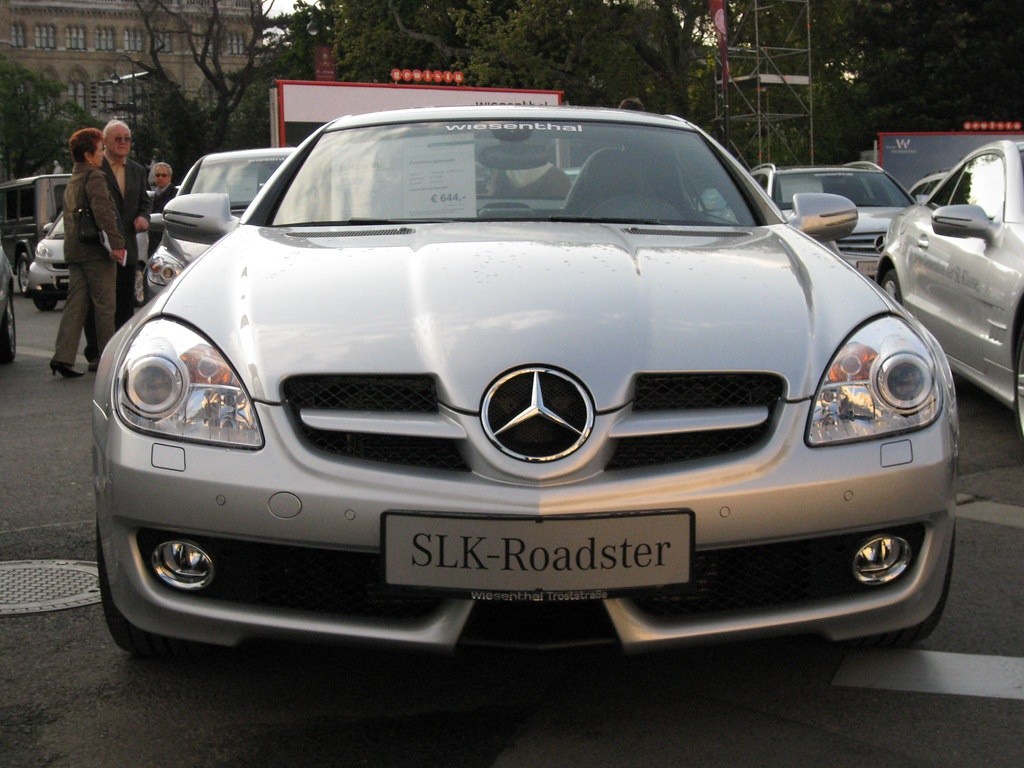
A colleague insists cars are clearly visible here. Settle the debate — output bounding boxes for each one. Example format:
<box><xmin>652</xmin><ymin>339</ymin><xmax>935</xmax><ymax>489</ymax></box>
<box><xmin>136</xmin><ymin>146</ymin><xmax>301</xmax><ymax>303</ymax></box>
<box><xmin>29</xmin><ymin>201</ymin><xmax>151</xmax><ymax>311</ymax></box>
<box><xmin>874</xmin><ymin>139</ymin><xmax>1023</xmax><ymax>431</ymax></box>
<box><xmin>904</xmin><ymin>171</ymin><xmax>949</xmax><ymax>203</ymax></box>
<box><xmin>87</xmin><ymin>107</ymin><xmax>959</xmax><ymax>670</ymax></box>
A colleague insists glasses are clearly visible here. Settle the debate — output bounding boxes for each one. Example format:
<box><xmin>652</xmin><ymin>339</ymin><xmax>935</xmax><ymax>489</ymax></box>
<box><xmin>156</xmin><ymin>174</ymin><xmax>171</xmax><ymax>177</ymax></box>
<box><xmin>107</xmin><ymin>137</ymin><xmax>132</xmax><ymax>142</ymax></box>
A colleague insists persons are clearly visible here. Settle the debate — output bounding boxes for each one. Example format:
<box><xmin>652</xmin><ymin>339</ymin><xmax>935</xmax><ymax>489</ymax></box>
<box><xmin>98</xmin><ymin>119</ymin><xmax>154</xmax><ymax>332</ymax></box>
<box><xmin>574</xmin><ymin>142</ymin><xmax>706</xmax><ymax>222</ymax></box>
<box><xmin>147</xmin><ymin>162</ymin><xmax>178</xmax><ymax>259</ymax></box>
<box><xmin>49</xmin><ymin>128</ymin><xmax>125</xmax><ymax>378</ymax></box>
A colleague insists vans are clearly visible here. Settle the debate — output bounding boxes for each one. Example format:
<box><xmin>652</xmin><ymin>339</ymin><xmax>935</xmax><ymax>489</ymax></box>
<box><xmin>0</xmin><ymin>175</ymin><xmax>76</xmax><ymax>295</ymax></box>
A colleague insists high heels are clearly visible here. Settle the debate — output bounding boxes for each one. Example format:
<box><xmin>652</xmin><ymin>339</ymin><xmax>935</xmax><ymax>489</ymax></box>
<box><xmin>50</xmin><ymin>360</ymin><xmax>85</xmax><ymax>378</ymax></box>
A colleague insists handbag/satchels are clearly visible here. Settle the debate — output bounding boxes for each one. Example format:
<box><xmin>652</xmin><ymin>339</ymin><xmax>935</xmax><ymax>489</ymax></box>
<box><xmin>74</xmin><ymin>171</ymin><xmax>125</xmax><ymax>238</ymax></box>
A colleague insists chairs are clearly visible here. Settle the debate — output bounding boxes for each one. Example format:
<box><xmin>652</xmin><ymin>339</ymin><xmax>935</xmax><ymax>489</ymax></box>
<box><xmin>560</xmin><ymin>145</ymin><xmax>695</xmax><ymax>223</ymax></box>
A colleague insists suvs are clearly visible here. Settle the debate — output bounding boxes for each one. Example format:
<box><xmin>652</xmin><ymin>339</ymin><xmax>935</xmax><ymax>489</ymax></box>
<box><xmin>750</xmin><ymin>161</ymin><xmax>920</xmax><ymax>286</ymax></box>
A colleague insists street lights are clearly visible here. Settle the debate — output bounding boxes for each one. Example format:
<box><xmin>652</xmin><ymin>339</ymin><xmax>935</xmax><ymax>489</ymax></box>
<box><xmin>111</xmin><ymin>55</ymin><xmax>137</xmax><ymax>150</ymax></box>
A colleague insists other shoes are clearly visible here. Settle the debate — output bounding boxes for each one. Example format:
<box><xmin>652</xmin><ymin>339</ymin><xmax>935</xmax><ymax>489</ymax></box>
<box><xmin>88</xmin><ymin>361</ymin><xmax>98</xmax><ymax>370</ymax></box>
